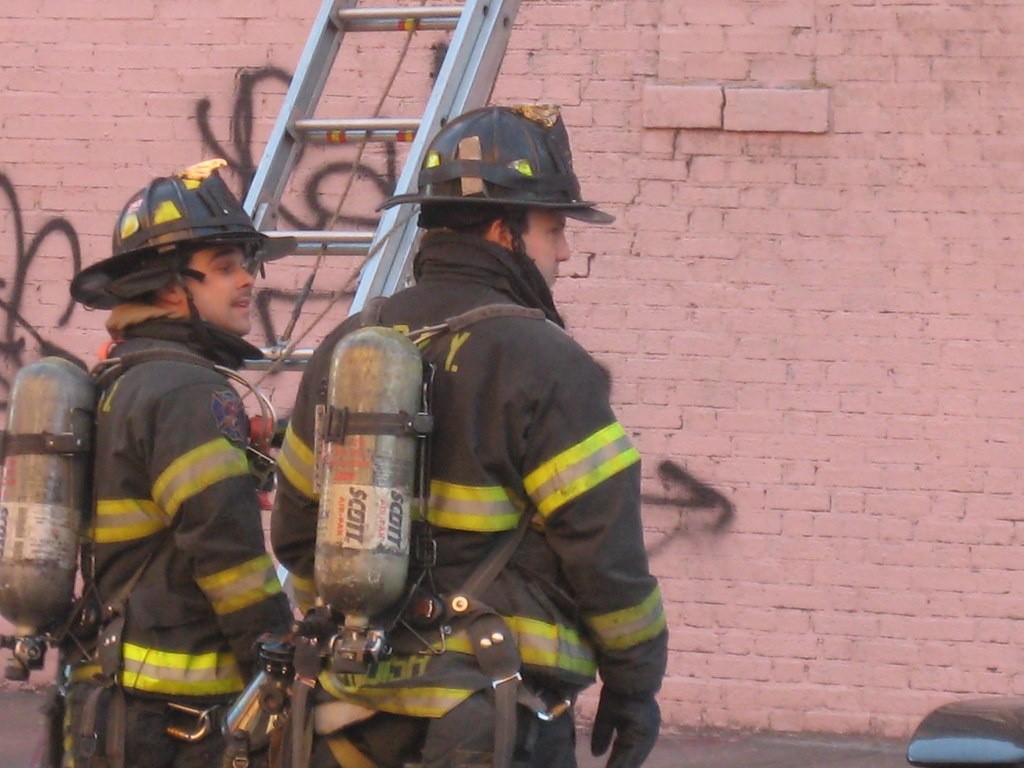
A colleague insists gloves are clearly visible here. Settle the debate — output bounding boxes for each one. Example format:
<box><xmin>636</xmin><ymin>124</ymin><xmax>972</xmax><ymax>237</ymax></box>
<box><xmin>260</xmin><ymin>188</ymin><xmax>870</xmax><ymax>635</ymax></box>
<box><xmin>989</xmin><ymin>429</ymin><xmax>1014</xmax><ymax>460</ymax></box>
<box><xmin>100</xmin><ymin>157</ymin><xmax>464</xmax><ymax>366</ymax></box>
<box><xmin>590</xmin><ymin>678</ymin><xmax>660</xmax><ymax>768</ymax></box>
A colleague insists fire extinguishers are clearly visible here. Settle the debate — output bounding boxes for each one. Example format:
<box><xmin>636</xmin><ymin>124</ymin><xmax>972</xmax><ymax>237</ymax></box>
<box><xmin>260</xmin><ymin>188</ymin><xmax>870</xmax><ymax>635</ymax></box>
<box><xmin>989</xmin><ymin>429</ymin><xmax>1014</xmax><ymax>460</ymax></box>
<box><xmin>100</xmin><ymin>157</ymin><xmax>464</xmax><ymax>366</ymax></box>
<box><xmin>313</xmin><ymin>326</ymin><xmax>426</xmax><ymax>663</ymax></box>
<box><xmin>0</xmin><ymin>357</ymin><xmax>90</xmax><ymax>682</ymax></box>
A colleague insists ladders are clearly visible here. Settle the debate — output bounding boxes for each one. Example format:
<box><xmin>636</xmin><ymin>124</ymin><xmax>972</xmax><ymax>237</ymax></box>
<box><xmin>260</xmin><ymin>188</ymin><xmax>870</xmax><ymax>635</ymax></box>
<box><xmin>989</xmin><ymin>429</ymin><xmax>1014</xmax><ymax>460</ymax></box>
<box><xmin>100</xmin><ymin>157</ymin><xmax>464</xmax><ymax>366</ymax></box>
<box><xmin>241</xmin><ymin>0</ymin><xmax>522</xmax><ymax>611</ymax></box>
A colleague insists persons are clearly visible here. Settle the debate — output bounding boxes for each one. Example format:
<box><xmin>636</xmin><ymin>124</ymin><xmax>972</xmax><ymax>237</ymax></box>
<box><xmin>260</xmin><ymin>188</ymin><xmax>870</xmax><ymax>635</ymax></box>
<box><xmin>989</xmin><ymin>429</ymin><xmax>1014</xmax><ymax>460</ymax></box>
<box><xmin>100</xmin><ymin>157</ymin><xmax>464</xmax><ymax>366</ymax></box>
<box><xmin>59</xmin><ymin>155</ymin><xmax>298</xmax><ymax>768</ymax></box>
<box><xmin>266</xmin><ymin>103</ymin><xmax>671</xmax><ymax>768</ymax></box>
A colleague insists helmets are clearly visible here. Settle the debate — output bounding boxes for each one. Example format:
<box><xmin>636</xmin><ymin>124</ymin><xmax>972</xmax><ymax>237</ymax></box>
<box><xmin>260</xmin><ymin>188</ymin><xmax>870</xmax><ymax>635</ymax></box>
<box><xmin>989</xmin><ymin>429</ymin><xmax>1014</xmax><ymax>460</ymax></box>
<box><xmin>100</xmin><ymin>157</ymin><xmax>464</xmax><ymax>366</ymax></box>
<box><xmin>68</xmin><ymin>157</ymin><xmax>298</xmax><ymax>308</ymax></box>
<box><xmin>374</xmin><ymin>103</ymin><xmax>616</xmax><ymax>226</ymax></box>
